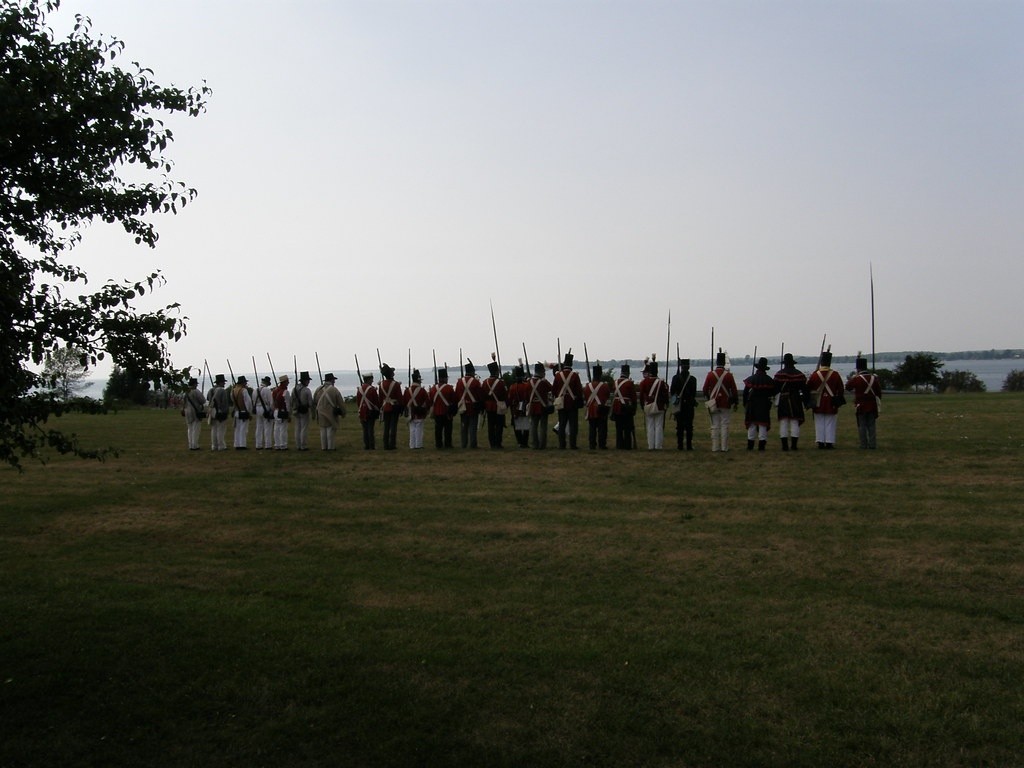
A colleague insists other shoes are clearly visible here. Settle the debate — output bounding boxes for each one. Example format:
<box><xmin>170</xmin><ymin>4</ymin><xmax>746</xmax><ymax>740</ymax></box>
<box><xmin>187</xmin><ymin>426</ymin><xmax>879</xmax><ymax>453</ymax></box>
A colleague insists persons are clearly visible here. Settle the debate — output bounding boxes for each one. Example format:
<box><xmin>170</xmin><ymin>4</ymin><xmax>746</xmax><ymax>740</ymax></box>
<box><xmin>845</xmin><ymin>358</ymin><xmax>882</xmax><ymax>448</ymax></box>
<box><xmin>357</xmin><ymin>363</ymin><xmax>552</xmax><ymax>450</ymax></box>
<box><xmin>314</xmin><ymin>373</ymin><xmax>346</xmax><ymax>450</ymax></box>
<box><xmin>207</xmin><ymin>374</ymin><xmax>229</xmax><ymax>451</ymax></box>
<box><xmin>252</xmin><ymin>376</ymin><xmax>273</xmax><ymax>450</ymax></box>
<box><xmin>772</xmin><ymin>353</ymin><xmax>807</xmax><ymax>451</ymax></box>
<box><xmin>552</xmin><ymin>354</ymin><xmax>584</xmax><ymax>449</ymax></box>
<box><xmin>291</xmin><ymin>371</ymin><xmax>315</xmax><ymax>449</ymax></box>
<box><xmin>183</xmin><ymin>378</ymin><xmax>206</xmax><ymax>450</ymax></box>
<box><xmin>807</xmin><ymin>352</ymin><xmax>844</xmax><ymax>449</ymax></box>
<box><xmin>742</xmin><ymin>356</ymin><xmax>773</xmax><ymax>450</ymax></box>
<box><xmin>703</xmin><ymin>352</ymin><xmax>739</xmax><ymax>452</ymax></box>
<box><xmin>271</xmin><ymin>375</ymin><xmax>291</xmax><ymax>451</ymax></box>
<box><xmin>670</xmin><ymin>359</ymin><xmax>697</xmax><ymax>450</ymax></box>
<box><xmin>639</xmin><ymin>362</ymin><xmax>669</xmax><ymax>450</ymax></box>
<box><xmin>610</xmin><ymin>364</ymin><xmax>637</xmax><ymax>449</ymax></box>
<box><xmin>231</xmin><ymin>376</ymin><xmax>252</xmax><ymax>449</ymax></box>
<box><xmin>584</xmin><ymin>366</ymin><xmax>610</xmax><ymax>449</ymax></box>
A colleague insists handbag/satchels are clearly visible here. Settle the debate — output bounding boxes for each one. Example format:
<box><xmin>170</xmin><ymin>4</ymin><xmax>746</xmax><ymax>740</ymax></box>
<box><xmin>195</xmin><ymin>393</ymin><xmax>882</xmax><ymax>424</ymax></box>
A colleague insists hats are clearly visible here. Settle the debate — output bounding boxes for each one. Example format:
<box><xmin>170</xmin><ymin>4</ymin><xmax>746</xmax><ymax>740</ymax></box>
<box><xmin>262</xmin><ymin>376</ymin><xmax>271</xmax><ymax>385</ymax></box>
<box><xmin>412</xmin><ymin>368</ymin><xmax>421</xmax><ymax>381</ymax></box>
<box><xmin>717</xmin><ymin>347</ymin><xmax>725</xmax><ymax>361</ymax></box>
<box><xmin>438</xmin><ymin>362</ymin><xmax>448</xmax><ymax>378</ymax></box>
<box><xmin>514</xmin><ymin>358</ymin><xmax>526</xmax><ymax>377</ymax></box>
<box><xmin>362</xmin><ymin>372</ymin><xmax>373</xmax><ymax>377</ymax></box>
<box><xmin>681</xmin><ymin>359</ymin><xmax>689</xmax><ymax>366</ymax></box>
<box><xmin>237</xmin><ymin>376</ymin><xmax>249</xmax><ymax>383</ymax></box>
<box><xmin>593</xmin><ymin>359</ymin><xmax>603</xmax><ymax>375</ymax></box>
<box><xmin>649</xmin><ymin>353</ymin><xmax>658</xmax><ymax>372</ymax></box>
<box><xmin>214</xmin><ymin>374</ymin><xmax>227</xmax><ymax>383</ymax></box>
<box><xmin>298</xmin><ymin>371</ymin><xmax>312</xmax><ymax>380</ymax></box>
<box><xmin>323</xmin><ymin>373</ymin><xmax>338</xmax><ymax>381</ymax></box>
<box><xmin>487</xmin><ymin>352</ymin><xmax>500</xmax><ymax>374</ymax></box>
<box><xmin>754</xmin><ymin>345</ymin><xmax>867</xmax><ymax>370</ymax></box>
<box><xmin>640</xmin><ymin>356</ymin><xmax>650</xmax><ymax>372</ymax></box>
<box><xmin>534</xmin><ymin>361</ymin><xmax>545</xmax><ymax>374</ymax></box>
<box><xmin>279</xmin><ymin>375</ymin><xmax>291</xmax><ymax>382</ymax></box>
<box><xmin>621</xmin><ymin>361</ymin><xmax>630</xmax><ymax>374</ymax></box>
<box><xmin>188</xmin><ymin>378</ymin><xmax>199</xmax><ymax>384</ymax></box>
<box><xmin>380</xmin><ymin>363</ymin><xmax>395</xmax><ymax>375</ymax></box>
<box><xmin>564</xmin><ymin>348</ymin><xmax>574</xmax><ymax>364</ymax></box>
<box><xmin>465</xmin><ymin>357</ymin><xmax>475</xmax><ymax>373</ymax></box>
